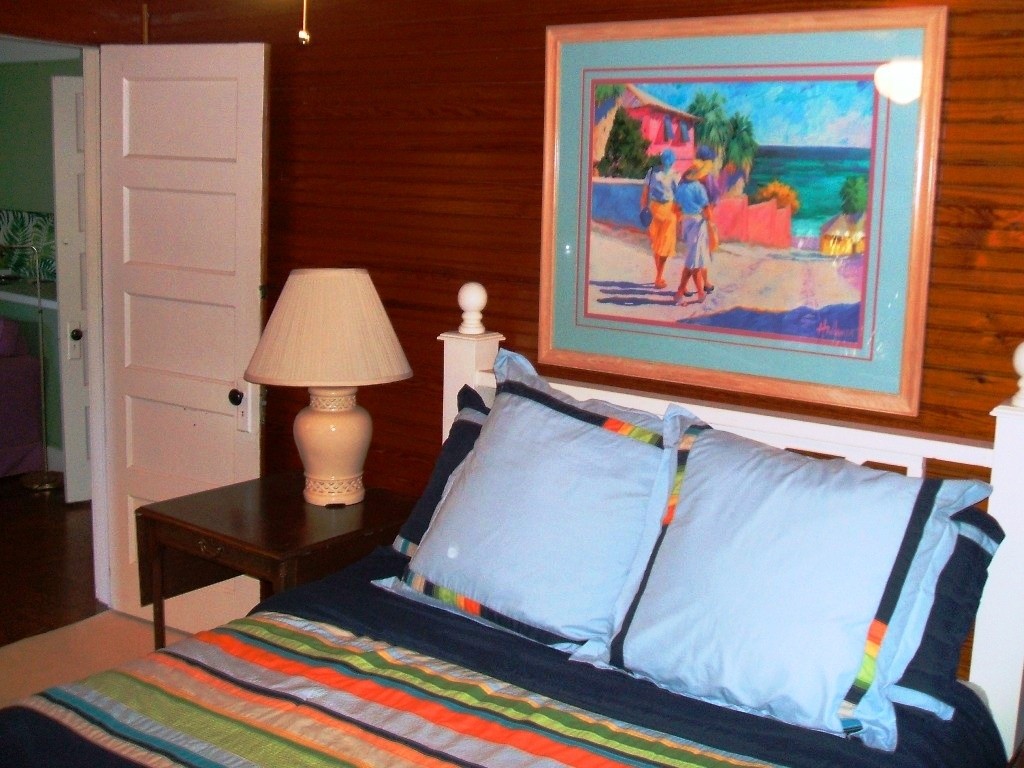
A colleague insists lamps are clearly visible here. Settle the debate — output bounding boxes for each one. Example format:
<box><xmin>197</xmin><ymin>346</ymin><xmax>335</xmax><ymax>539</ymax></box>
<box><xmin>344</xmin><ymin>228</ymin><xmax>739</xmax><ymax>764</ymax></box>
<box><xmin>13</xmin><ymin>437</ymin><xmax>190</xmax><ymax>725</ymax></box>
<box><xmin>245</xmin><ymin>267</ymin><xmax>409</xmax><ymax>505</ymax></box>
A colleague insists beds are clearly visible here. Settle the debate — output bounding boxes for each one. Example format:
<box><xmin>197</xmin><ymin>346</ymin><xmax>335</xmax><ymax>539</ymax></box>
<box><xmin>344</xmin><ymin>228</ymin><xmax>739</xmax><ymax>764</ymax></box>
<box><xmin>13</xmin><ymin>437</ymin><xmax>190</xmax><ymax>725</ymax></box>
<box><xmin>1</xmin><ymin>284</ymin><xmax>1024</xmax><ymax>767</ymax></box>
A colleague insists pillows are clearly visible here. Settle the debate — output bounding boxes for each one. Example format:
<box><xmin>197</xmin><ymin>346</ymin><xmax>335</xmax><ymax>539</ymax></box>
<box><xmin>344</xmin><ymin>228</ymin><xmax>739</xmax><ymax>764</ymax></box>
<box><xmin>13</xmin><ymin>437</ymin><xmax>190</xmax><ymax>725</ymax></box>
<box><xmin>370</xmin><ymin>349</ymin><xmax>1005</xmax><ymax>751</ymax></box>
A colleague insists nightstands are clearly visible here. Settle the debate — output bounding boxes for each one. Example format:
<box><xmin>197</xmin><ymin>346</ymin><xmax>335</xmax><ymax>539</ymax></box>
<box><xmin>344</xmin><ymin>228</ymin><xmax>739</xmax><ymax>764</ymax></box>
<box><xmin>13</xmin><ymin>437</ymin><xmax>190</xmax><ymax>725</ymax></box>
<box><xmin>135</xmin><ymin>482</ymin><xmax>427</xmax><ymax>660</ymax></box>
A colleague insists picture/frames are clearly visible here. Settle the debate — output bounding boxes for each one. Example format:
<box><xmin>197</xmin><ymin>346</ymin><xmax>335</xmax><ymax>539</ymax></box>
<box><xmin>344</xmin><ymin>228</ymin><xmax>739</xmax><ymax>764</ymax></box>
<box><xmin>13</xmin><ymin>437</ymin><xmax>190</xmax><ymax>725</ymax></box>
<box><xmin>537</xmin><ymin>6</ymin><xmax>949</xmax><ymax>417</ymax></box>
